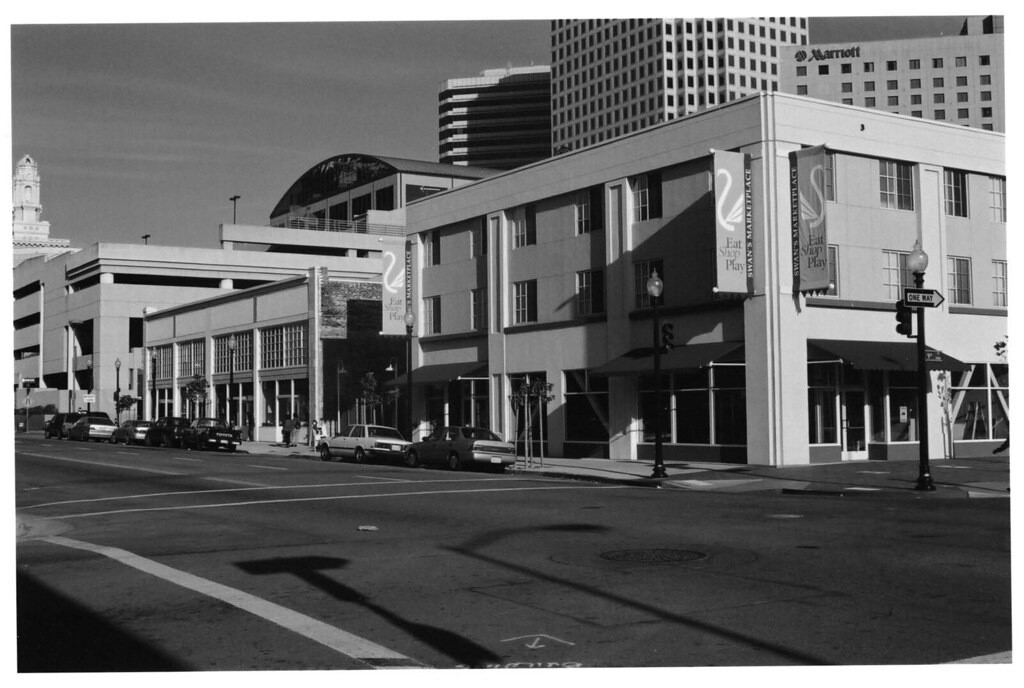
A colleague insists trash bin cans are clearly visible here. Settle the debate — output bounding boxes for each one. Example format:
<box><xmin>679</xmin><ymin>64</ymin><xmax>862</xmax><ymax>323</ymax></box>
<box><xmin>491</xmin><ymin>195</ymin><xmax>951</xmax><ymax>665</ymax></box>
<box><xmin>17</xmin><ymin>418</ymin><xmax>25</xmax><ymax>431</ymax></box>
<box><xmin>238</xmin><ymin>426</ymin><xmax>250</xmax><ymax>441</ymax></box>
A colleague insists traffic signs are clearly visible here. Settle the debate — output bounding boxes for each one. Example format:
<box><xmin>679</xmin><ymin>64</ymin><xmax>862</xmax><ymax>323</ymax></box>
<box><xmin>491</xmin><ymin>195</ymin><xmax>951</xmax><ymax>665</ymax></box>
<box><xmin>904</xmin><ymin>288</ymin><xmax>944</xmax><ymax>307</ymax></box>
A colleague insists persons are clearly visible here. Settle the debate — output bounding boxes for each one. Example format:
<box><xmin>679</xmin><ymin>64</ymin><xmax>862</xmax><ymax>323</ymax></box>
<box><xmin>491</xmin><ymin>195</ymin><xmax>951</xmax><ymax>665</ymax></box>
<box><xmin>311</xmin><ymin>418</ymin><xmax>327</xmax><ymax>453</ymax></box>
<box><xmin>247</xmin><ymin>412</ymin><xmax>254</xmax><ymax>441</ymax></box>
<box><xmin>115</xmin><ymin>418</ymin><xmax>118</xmax><ymax>426</ymax></box>
<box><xmin>283</xmin><ymin>413</ymin><xmax>301</xmax><ymax>447</ymax></box>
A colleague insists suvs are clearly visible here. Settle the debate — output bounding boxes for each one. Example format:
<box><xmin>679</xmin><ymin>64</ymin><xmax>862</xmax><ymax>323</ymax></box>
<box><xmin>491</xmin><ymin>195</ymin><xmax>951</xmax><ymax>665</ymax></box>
<box><xmin>45</xmin><ymin>413</ymin><xmax>82</xmax><ymax>440</ymax></box>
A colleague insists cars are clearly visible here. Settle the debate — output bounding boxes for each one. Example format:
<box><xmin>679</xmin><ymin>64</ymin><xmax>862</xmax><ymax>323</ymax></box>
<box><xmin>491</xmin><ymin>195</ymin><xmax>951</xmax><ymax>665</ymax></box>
<box><xmin>110</xmin><ymin>420</ymin><xmax>155</xmax><ymax>445</ymax></box>
<box><xmin>67</xmin><ymin>416</ymin><xmax>119</xmax><ymax>443</ymax></box>
<box><xmin>320</xmin><ymin>424</ymin><xmax>412</xmax><ymax>464</ymax></box>
<box><xmin>404</xmin><ymin>424</ymin><xmax>517</xmax><ymax>473</ymax></box>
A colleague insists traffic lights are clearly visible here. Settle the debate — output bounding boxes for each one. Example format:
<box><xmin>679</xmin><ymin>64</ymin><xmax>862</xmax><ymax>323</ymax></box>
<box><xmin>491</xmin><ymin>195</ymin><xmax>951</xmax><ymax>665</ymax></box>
<box><xmin>897</xmin><ymin>299</ymin><xmax>912</xmax><ymax>334</ymax></box>
<box><xmin>659</xmin><ymin>322</ymin><xmax>674</xmax><ymax>351</ymax></box>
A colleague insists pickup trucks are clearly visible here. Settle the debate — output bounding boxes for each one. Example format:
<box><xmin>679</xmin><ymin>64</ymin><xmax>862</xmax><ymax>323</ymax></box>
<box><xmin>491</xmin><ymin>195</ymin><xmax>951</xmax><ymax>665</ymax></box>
<box><xmin>181</xmin><ymin>418</ymin><xmax>243</xmax><ymax>452</ymax></box>
<box><xmin>145</xmin><ymin>416</ymin><xmax>192</xmax><ymax>449</ymax></box>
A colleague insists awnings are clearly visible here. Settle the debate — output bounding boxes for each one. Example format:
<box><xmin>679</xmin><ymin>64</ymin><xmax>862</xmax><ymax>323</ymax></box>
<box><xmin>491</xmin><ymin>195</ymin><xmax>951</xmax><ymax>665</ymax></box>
<box><xmin>384</xmin><ymin>362</ymin><xmax>487</xmax><ymax>386</ymax></box>
<box><xmin>590</xmin><ymin>340</ymin><xmax>744</xmax><ymax>375</ymax></box>
<box><xmin>807</xmin><ymin>339</ymin><xmax>973</xmax><ymax>372</ymax></box>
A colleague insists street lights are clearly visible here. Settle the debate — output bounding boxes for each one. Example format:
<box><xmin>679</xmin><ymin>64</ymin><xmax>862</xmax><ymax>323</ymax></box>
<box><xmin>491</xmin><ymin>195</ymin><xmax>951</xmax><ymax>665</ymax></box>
<box><xmin>115</xmin><ymin>358</ymin><xmax>121</xmax><ymax>429</ymax></box>
<box><xmin>385</xmin><ymin>356</ymin><xmax>398</xmax><ymax>429</ymax></box>
<box><xmin>228</xmin><ymin>334</ymin><xmax>236</xmax><ymax>434</ymax></box>
<box><xmin>647</xmin><ymin>268</ymin><xmax>668</xmax><ymax>479</ymax></box>
<box><xmin>337</xmin><ymin>360</ymin><xmax>347</xmax><ymax>436</ymax></box>
<box><xmin>907</xmin><ymin>240</ymin><xmax>937</xmax><ymax>492</ymax></box>
<box><xmin>86</xmin><ymin>359</ymin><xmax>91</xmax><ymax>417</ymax></box>
<box><xmin>404</xmin><ymin>307</ymin><xmax>416</xmax><ymax>443</ymax></box>
